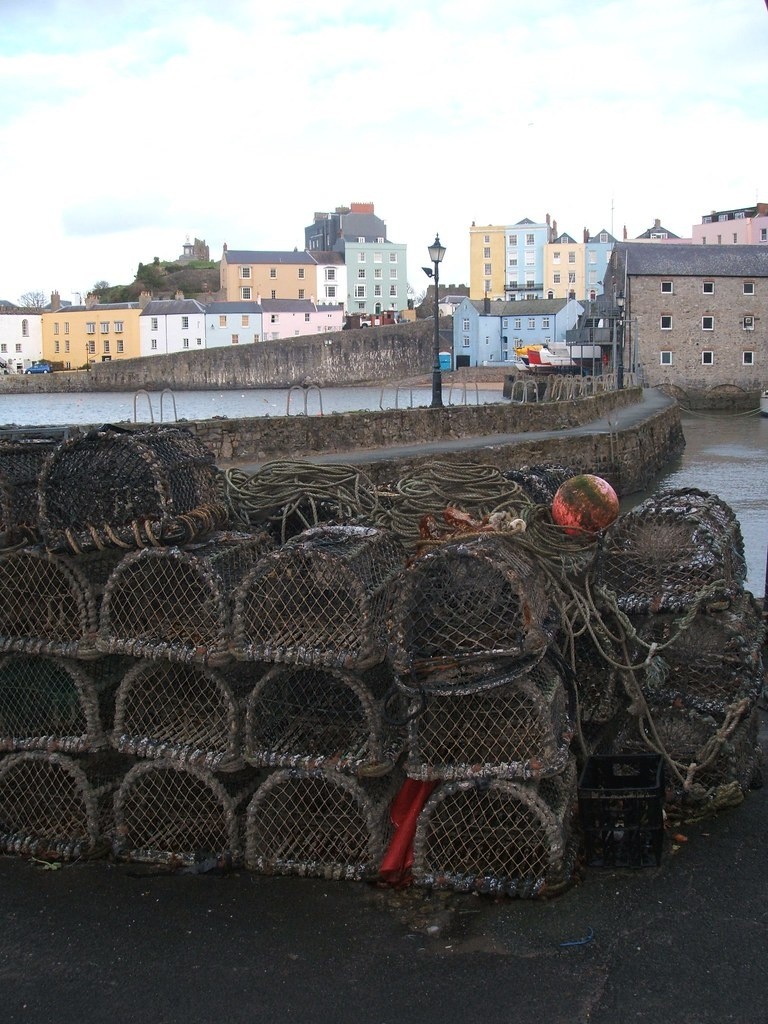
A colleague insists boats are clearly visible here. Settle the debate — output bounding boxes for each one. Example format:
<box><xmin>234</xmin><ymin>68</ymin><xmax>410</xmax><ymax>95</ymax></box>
<box><xmin>759</xmin><ymin>390</ymin><xmax>768</xmax><ymax>414</ymax></box>
<box><xmin>515</xmin><ymin>342</ymin><xmax>601</xmax><ymax>375</ymax></box>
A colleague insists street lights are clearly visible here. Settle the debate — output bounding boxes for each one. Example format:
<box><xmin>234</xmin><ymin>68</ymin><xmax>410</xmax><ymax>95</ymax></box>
<box><xmin>86</xmin><ymin>343</ymin><xmax>89</xmax><ymax>371</ymax></box>
<box><xmin>615</xmin><ymin>289</ymin><xmax>626</xmax><ymax>389</ymax></box>
<box><xmin>422</xmin><ymin>233</ymin><xmax>446</xmax><ymax>407</ymax></box>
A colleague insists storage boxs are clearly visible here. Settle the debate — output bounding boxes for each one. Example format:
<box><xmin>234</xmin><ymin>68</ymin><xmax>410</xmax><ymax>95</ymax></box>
<box><xmin>577</xmin><ymin>750</ymin><xmax>667</xmax><ymax>870</ymax></box>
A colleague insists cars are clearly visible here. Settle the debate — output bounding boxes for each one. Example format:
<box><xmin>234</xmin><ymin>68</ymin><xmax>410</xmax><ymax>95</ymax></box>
<box><xmin>25</xmin><ymin>362</ymin><xmax>53</xmax><ymax>374</ymax></box>
<box><xmin>360</xmin><ymin>314</ymin><xmax>397</xmax><ymax>328</ymax></box>
